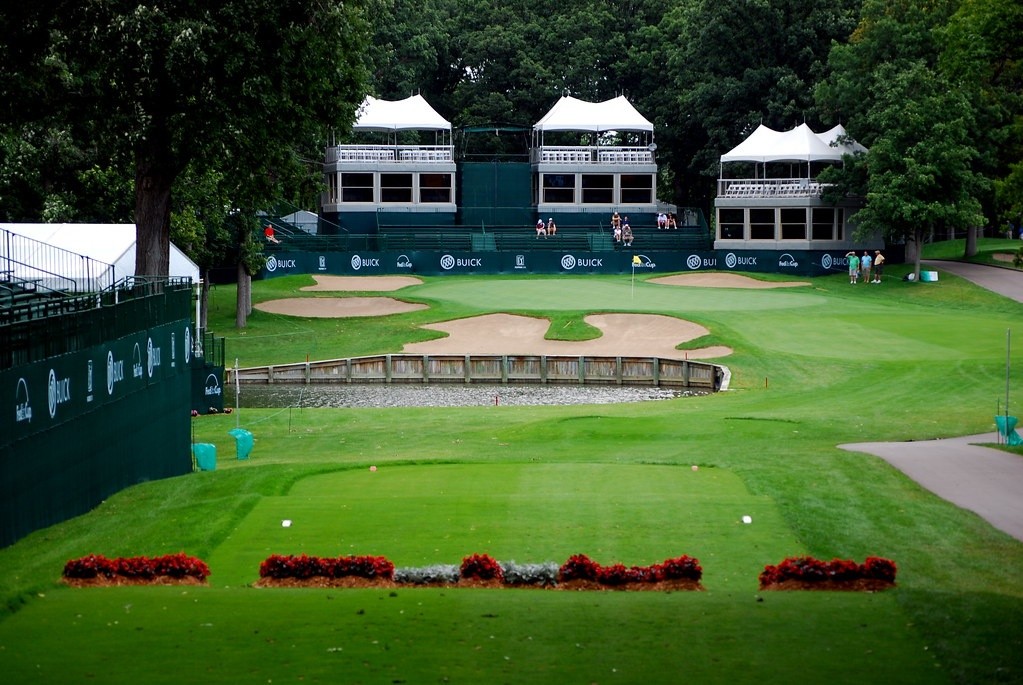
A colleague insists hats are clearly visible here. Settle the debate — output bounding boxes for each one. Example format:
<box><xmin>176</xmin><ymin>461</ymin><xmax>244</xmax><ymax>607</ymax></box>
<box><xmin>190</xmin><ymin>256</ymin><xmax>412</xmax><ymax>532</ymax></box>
<box><xmin>549</xmin><ymin>217</ymin><xmax>553</xmax><ymax>220</ymax></box>
<box><xmin>538</xmin><ymin>219</ymin><xmax>542</xmax><ymax>224</ymax></box>
<box><xmin>659</xmin><ymin>212</ymin><xmax>663</xmax><ymax>215</ymax></box>
<box><xmin>625</xmin><ymin>224</ymin><xmax>629</xmax><ymax>226</ymax></box>
<box><xmin>614</xmin><ymin>211</ymin><xmax>618</xmax><ymax>215</ymax></box>
<box><xmin>875</xmin><ymin>249</ymin><xmax>880</xmax><ymax>253</ymax></box>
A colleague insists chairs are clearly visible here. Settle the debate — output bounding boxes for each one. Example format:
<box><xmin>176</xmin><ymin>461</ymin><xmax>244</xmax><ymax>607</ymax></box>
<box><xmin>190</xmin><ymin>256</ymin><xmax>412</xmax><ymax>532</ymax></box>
<box><xmin>536</xmin><ymin>150</ymin><xmax>652</xmax><ymax>164</ymax></box>
<box><xmin>331</xmin><ymin>150</ymin><xmax>451</xmax><ymax>162</ymax></box>
<box><xmin>725</xmin><ymin>182</ymin><xmax>823</xmax><ymax>197</ymax></box>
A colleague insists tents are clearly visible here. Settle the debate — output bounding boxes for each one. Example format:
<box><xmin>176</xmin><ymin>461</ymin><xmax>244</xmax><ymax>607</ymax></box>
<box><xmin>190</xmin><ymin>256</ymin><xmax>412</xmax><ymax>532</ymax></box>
<box><xmin>719</xmin><ymin>122</ymin><xmax>871</xmax><ymax>183</ymax></box>
<box><xmin>339</xmin><ymin>95</ymin><xmax>453</xmax><ymax>150</ymax></box>
<box><xmin>532</xmin><ymin>95</ymin><xmax>653</xmax><ymax>162</ymax></box>
<box><xmin>0</xmin><ymin>222</ymin><xmax>201</xmax><ymax>329</ymax></box>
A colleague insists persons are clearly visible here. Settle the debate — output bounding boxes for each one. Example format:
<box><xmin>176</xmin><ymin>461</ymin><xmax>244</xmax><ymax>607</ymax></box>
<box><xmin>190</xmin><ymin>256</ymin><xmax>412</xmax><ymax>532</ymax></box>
<box><xmin>657</xmin><ymin>212</ymin><xmax>678</xmax><ymax>229</ymax></box>
<box><xmin>614</xmin><ymin>226</ymin><xmax>622</xmax><ymax>242</ymax></box>
<box><xmin>610</xmin><ymin>212</ymin><xmax>622</xmax><ymax>229</ymax></box>
<box><xmin>265</xmin><ymin>224</ymin><xmax>282</xmax><ymax>245</ymax></box>
<box><xmin>536</xmin><ymin>219</ymin><xmax>547</xmax><ymax>239</ymax></box>
<box><xmin>861</xmin><ymin>251</ymin><xmax>873</xmax><ymax>283</ymax></box>
<box><xmin>622</xmin><ymin>217</ymin><xmax>630</xmax><ymax>228</ymax></box>
<box><xmin>871</xmin><ymin>250</ymin><xmax>885</xmax><ymax>283</ymax></box>
<box><xmin>547</xmin><ymin>218</ymin><xmax>557</xmax><ymax>235</ymax></box>
<box><xmin>845</xmin><ymin>252</ymin><xmax>860</xmax><ymax>284</ymax></box>
<box><xmin>621</xmin><ymin>224</ymin><xmax>634</xmax><ymax>246</ymax></box>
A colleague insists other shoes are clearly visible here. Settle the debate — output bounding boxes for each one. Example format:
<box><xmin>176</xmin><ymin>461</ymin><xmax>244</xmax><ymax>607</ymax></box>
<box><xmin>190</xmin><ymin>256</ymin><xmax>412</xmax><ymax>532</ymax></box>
<box><xmin>544</xmin><ymin>237</ymin><xmax>547</xmax><ymax>239</ymax></box>
<box><xmin>870</xmin><ymin>279</ymin><xmax>877</xmax><ymax>283</ymax></box>
<box><xmin>853</xmin><ymin>282</ymin><xmax>857</xmax><ymax>285</ymax></box>
<box><xmin>876</xmin><ymin>279</ymin><xmax>882</xmax><ymax>283</ymax></box>
<box><xmin>667</xmin><ymin>227</ymin><xmax>669</xmax><ymax>229</ymax></box>
<box><xmin>675</xmin><ymin>227</ymin><xmax>678</xmax><ymax>229</ymax></box>
<box><xmin>619</xmin><ymin>227</ymin><xmax>621</xmax><ymax>229</ymax></box>
<box><xmin>623</xmin><ymin>243</ymin><xmax>626</xmax><ymax>246</ymax></box>
<box><xmin>850</xmin><ymin>280</ymin><xmax>853</xmax><ymax>285</ymax></box>
<box><xmin>536</xmin><ymin>237</ymin><xmax>538</xmax><ymax>239</ymax></box>
<box><xmin>627</xmin><ymin>243</ymin><xmax>631</xmax><ymax>246</ymax></box>
<box><xmin>613</xmin><ymin>226</ymin><xmax>615</xmax><ymax>229</ymax></box>
<box><xmin>278</xmin><ymin>240</ymin><xmax>282</xmax><ymax>245</ymax></box>
<box><xmin>658</xmin><ymin>227</ymin><xmax>661</xmax><ymax>229</ymax></box>
<box><xmin>665</xmin><ymin>227</ymin><xmax>667</xmax><ymax>229</ymax></box>
